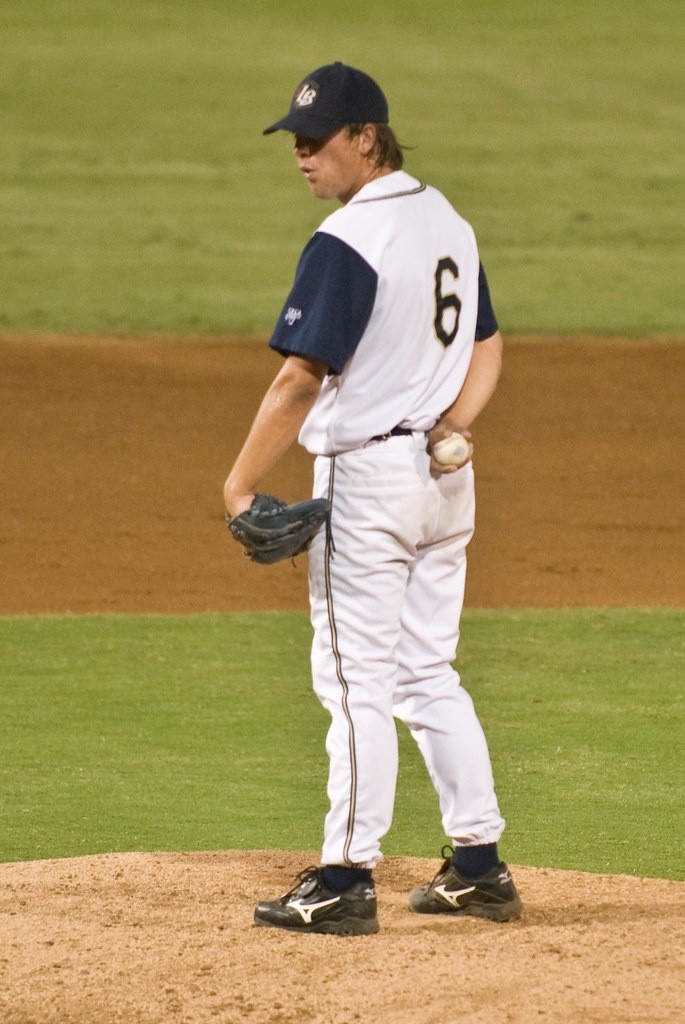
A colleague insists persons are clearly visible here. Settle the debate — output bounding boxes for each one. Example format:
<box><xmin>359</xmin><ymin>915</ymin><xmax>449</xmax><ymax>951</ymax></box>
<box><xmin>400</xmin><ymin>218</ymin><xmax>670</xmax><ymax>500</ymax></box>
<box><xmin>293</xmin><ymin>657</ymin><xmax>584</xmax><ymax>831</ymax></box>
<box><xmin>222</xmin><ymin>61</ymin><xmax>523</xmax><ymax>936</ymax></box>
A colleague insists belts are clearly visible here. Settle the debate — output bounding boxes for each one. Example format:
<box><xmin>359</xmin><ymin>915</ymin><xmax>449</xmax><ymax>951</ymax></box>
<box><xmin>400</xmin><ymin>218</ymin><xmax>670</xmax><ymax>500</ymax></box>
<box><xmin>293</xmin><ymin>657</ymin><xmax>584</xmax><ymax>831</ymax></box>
<box><xmin>372</xmin><ymin>425</ymin><xmax>416</xmax><ymax>441</ymax></box>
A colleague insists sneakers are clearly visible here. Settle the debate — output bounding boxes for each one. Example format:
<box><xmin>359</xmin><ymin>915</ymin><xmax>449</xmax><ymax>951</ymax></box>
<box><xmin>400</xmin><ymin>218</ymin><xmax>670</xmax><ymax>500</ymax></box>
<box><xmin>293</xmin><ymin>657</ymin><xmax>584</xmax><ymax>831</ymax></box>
<box><xmin>408</xmin><ymin>844</ymin><xmax>524</xmax><ymax>922</ymax></box>
<box><xmin>253</xmin><ymin>865</ymin><xmax>381</xmax><ymax>935</ymax></box>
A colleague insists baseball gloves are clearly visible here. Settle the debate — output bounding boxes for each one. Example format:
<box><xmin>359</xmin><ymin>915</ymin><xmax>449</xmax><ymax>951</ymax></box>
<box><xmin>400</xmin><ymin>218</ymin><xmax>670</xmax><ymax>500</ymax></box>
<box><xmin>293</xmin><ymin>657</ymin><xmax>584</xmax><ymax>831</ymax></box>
<box><xmin>224</xmin><ymin>492</ymin><xmax>331</xmax><ymax>569</ymax></box>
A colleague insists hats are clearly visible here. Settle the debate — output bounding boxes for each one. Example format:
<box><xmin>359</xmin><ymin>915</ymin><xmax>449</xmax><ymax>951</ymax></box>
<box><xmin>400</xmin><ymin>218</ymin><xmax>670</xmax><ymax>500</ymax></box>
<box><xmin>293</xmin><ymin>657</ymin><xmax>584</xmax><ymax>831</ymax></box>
<box><xmin>263</xmin><ymin>61</ymin><xmax>389</xmax><ymax>138</ymax></box>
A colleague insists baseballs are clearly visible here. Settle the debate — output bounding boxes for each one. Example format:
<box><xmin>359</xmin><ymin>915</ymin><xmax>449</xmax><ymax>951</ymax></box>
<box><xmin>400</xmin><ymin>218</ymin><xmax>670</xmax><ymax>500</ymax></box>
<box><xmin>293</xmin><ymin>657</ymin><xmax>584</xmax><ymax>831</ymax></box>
<box><xmin>433</xmin><ymin>431</ymin><xmax>470</xmax><ymax>466</ymax></box>
